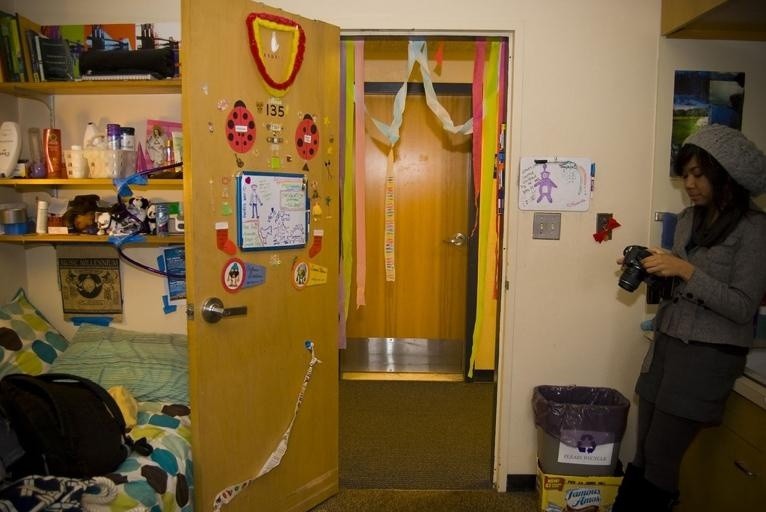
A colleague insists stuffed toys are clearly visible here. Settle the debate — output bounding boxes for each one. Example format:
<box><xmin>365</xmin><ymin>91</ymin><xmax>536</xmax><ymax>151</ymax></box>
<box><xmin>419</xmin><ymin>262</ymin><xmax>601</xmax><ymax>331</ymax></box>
<box><xmin>95</xmin><ymin>193</ymin><xmax>156</xmax><ymax>236</ymax></box>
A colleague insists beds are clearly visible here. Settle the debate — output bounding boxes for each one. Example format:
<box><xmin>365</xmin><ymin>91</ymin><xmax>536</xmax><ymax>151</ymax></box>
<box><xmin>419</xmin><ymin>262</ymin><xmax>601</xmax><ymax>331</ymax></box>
<box><xmin>0</xmin><ymin>335</ymin><xmax>197</xmax><ymax>511</ymax></box>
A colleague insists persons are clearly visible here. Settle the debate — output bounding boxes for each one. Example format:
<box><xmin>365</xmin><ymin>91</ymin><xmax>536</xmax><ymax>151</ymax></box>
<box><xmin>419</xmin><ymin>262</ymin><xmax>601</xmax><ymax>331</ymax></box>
<box><xmin>611</xmin><ymin>121</ymin><xmax>764</xmax><ymax>508</ymax></box>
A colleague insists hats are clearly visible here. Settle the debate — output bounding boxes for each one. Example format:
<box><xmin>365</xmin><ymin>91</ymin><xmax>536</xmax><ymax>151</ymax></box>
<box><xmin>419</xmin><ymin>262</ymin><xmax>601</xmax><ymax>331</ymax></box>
<box><xmin>682</xmin><ymin>124</ymin><xmax>762</xmax><ymax>196</ymax></box>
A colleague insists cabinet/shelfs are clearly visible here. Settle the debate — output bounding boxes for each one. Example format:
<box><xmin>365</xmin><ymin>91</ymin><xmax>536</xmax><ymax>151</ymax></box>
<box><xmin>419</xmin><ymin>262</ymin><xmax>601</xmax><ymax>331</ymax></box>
<box><xmin>0</xmin><ymin>79</ymin><xmax>185</xmax><ymax>245</ymax></box>
<box><xmin>680</xmin><ymin>378</ymin><xmax>765</xmax><ymax>511</ymax></box>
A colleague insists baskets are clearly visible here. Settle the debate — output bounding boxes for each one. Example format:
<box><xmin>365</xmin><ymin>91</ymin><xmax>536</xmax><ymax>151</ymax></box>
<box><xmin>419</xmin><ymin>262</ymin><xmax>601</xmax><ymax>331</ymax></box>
<box><xmin>65</xmin><ymin>147</ymin><xmax>137</xmax><ymax>178</ymax></box>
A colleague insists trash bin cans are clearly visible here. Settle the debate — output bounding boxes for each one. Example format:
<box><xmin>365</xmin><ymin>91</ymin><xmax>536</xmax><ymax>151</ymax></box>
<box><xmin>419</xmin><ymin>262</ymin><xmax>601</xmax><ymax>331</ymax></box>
<box><xmin>532</xmin><ymin>384</ymin><xmax>630</xmax><ymax>477</ymax></box>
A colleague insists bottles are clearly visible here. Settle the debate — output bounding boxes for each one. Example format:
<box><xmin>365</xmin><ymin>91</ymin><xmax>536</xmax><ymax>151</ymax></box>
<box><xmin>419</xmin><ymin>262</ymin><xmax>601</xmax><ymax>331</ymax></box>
<box><xmin>45</xmin><ymin>128</ymin><xmax>62</xmax><ymax>179</ymax></box>
<box><xmin>28</xmin><ymin>127</ymin><xmax>45</xmax><ymax>179</ymax></box>
<box><xmin>106</xmin><ymin>124</ymin><xmax>121</xmax><ymax>152</ymax></box>
<box><xmin>35</xmin><ymin>201</ymin><xmax>49</xmax><ymax>234</ymax></box>
<box><xmin>154</xmin><ymin>201</ymin><xmax>169</xmax><ymax>238</ymax></box>
<box><xmin>121</xmin><ymin>127</ymin><xmax>135</xmax><ymax>150</ymax></box>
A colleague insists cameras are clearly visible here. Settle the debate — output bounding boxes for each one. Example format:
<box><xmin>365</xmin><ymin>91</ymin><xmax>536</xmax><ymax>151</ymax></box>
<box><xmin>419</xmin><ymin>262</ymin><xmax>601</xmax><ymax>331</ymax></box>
<box><xmin>618</xmin><ymin>245</ymin><xmax>658</xmax><ymax>293</ymax></box>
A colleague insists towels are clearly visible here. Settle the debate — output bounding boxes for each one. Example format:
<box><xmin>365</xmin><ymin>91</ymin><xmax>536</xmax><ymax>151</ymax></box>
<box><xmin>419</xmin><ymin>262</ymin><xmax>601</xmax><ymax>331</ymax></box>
<box><xmin>661</xmin><ymin>212</ymin><xmax>678</xmax><ymax>248</ymax></box>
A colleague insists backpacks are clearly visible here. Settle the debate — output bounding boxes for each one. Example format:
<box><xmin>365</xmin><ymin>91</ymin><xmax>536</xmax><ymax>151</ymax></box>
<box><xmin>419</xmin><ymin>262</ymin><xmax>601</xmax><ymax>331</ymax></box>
<box><xmin>0</xmin><ymin>373</ymin><xmax>127</xmax><ymax>477</ymax></box>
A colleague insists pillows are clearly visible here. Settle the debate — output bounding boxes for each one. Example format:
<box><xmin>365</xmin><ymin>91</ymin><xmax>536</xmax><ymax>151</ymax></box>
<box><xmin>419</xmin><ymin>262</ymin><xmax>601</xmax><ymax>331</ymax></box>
<box><xmin>60</xmin><ymin>321</ymin><xmax>191</xmax><ymax>408</ymax></box>
<box><xmin>0</xmin><ymin>288</ymin><xmax>63</xmax><ymax>396</ymax></box>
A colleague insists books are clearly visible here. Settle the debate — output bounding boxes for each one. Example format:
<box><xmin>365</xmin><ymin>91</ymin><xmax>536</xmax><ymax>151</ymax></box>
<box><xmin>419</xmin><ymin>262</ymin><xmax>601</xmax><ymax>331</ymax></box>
<box><xmin>1</xmin><ymin>9</ymin><xmax>73</xmax><ymax>82</ymax></box>
<box><xmin>81</xmin><ymin>74</ymin><xmax>158</xmax><ymax>82</ymax></box>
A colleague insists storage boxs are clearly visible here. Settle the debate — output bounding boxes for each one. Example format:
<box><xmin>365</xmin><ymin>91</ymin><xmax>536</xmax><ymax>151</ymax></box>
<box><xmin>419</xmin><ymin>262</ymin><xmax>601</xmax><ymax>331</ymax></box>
<box><xmin>533</xmin><ymin>459</ymin><xmax>625</xmax><ymax>512</ymax></box>
